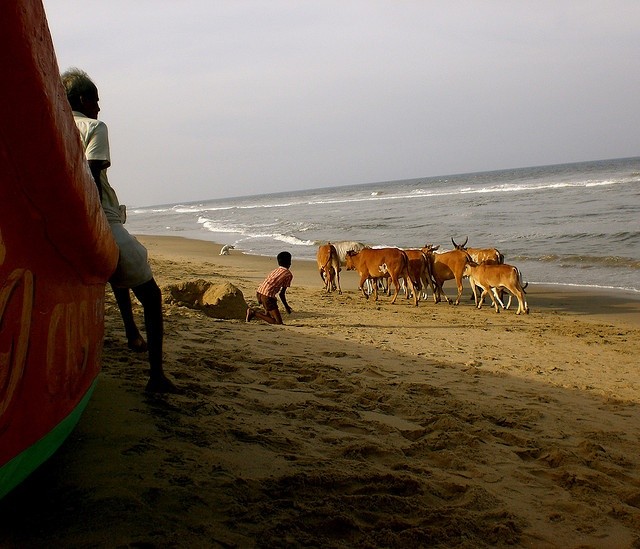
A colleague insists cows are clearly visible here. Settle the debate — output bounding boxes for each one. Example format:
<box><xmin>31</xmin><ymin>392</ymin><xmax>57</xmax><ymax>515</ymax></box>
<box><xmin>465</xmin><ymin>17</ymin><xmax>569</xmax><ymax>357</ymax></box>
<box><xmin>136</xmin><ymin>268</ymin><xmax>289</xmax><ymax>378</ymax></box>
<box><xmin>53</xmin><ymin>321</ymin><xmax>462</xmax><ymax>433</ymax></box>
<box><xmin>345</xmin><ymin>247</ymin><xmax>420</xmax><ymax>307</ymax></box>
<box><xmin>462</xmin><ymin>260</ymin><xmax>528</xmax><ymax>315</ymax></box>
<box><xmin>451</xmin><ymin>235</ymin><xmax>507</xmax><ymax>306</ymax></box>
<box><xmin>469</xmin><ymin>259</ymin><xmax>530</xmax><ymax>310</ymax></box>
<box><xmin>366</xmin><ymin>278</ymin><xmax>387</xmax><ymax>295</ymax></box>
<box><xmin>324</xmin><ymin>239</ymin><xmax>366</xmax><ymax>291</ymax></box>
<box><xmin>317</xmin><ymin>241</ymin><xmax>344</xmax><ymax>295</ymax></box>
<box><xmin>421</xmin><ymin>243</ymin><xmax>479</xmax><ymax>308</ymax></box>
<box><xmin>386</xmin><ymin>248</ymin><xmax>440</xmax><ymax>304</ymax></box>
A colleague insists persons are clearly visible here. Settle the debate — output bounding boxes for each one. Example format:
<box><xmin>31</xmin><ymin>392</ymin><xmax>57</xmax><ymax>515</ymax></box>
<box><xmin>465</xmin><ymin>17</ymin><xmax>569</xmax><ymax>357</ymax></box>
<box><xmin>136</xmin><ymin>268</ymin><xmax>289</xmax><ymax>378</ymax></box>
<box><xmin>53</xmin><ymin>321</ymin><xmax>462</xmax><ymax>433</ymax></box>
<box><xmin>60</xmin><ymin>68</ymin><xmax>182</xmax><ymax>394</ymax></box>
<box><xmin>245</xmin><ymin>251</ymin><xmax>293</xmax><ymax>325</ymax></box>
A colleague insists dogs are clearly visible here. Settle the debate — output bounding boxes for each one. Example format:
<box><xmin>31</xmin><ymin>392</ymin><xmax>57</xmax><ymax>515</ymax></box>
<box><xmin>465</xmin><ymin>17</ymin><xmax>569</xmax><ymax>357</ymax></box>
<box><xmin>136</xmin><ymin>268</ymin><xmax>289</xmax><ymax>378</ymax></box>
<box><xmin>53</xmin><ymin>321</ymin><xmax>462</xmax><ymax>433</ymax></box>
<box><xmin>219</xmin><ymin>244</ymin><xmax>236</xmax><ymax>256</ymax></box>
